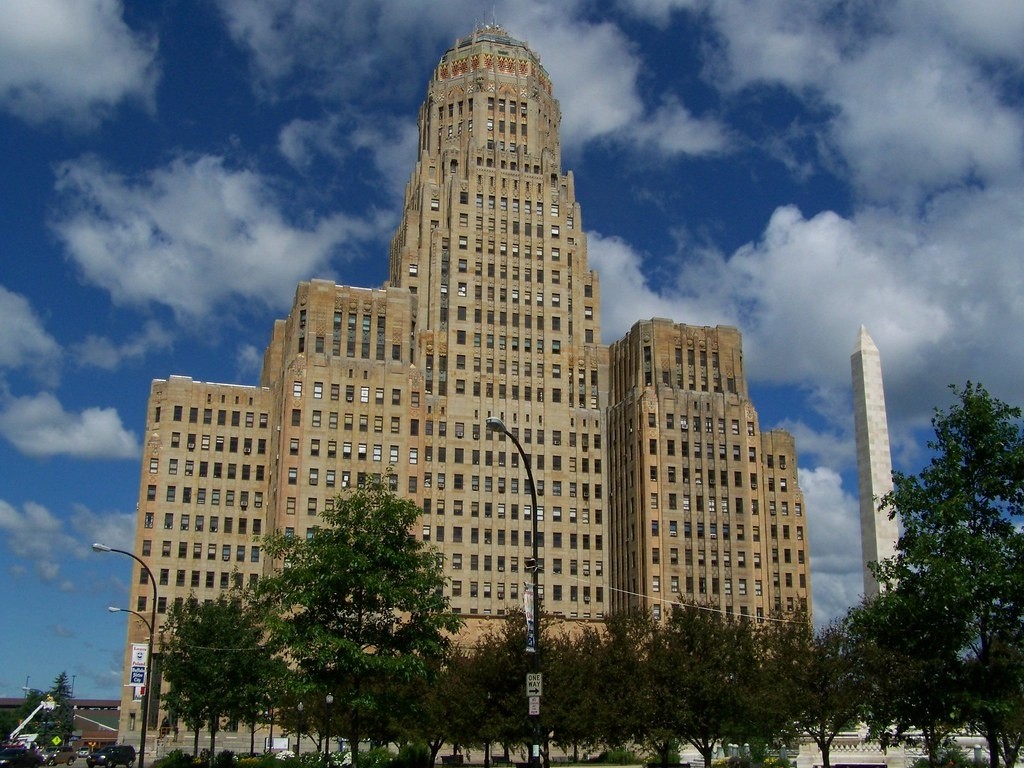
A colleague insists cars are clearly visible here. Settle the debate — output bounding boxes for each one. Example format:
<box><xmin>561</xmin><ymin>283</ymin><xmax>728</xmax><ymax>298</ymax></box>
<box><xmin>77</xmin><ymin>747</ymin><xmax>90</xmax><ymax>757</ymax></box>
<box><xmin>0</xmin><ymin>749</ymin><xmax>39</xmax><ymax>768</ymax></box>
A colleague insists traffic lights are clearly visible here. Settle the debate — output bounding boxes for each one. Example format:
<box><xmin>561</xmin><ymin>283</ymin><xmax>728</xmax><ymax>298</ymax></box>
<box><xmin>57</xmin><ymin>721</ymin><xmax>60</xmax><ymax>728</ymax></box>
<box><xmin>35</xmin><ymin>721</ymin><xmax>38</xmax><ymax>729</ymax></box>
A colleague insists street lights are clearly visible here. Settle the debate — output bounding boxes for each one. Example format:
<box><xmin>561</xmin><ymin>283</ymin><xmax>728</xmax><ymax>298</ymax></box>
<box><xmin>296</xmin><ymin>701</ymin><xmax>304</xmax><ymax>762</ymax></box>
<box><xmin>22</xmin><ymin>687</ymin><xmax>48</xmax><ymax>745</ymax></box>
<box><xmin>92</xmin><ymin>542</ymin><xmax>157</xmax><ymax>768</ymax></box>
<box><xmin>25</xmin><ymin>675</ymin><xmax>31</xmax><ymax>703</ymax></box>
<box><xmin>483</xmin><ymin>414</ymin><xmax>543</xmax><ymax>768</ymax></box>
<box><xmin>325</xmin><ymin>692</ymin><xmax>334</xmax><ymax>767</ymax></box>
<box><xmin>71</xmin><ymin>675</ymin><xmax>77</xmax><ymax>701</ymax></box>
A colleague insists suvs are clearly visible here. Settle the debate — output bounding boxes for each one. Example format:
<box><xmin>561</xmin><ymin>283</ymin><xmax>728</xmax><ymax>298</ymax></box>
<box><xmin>38</xmin><ymin>746</ymin><xmax>77</xmax><ymax>766</ymax></box>
<box><xmin>84</xmin><ymin>745</ymin><xmax>137</xmax><ymax>768</ymax></box>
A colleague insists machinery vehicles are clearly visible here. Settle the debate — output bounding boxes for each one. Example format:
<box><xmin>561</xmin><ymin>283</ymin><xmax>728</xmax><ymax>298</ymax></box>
<box><xmin>0</xmin><ymin>694</ymin><xmax>61</xmax><ymax>752</ymax></box>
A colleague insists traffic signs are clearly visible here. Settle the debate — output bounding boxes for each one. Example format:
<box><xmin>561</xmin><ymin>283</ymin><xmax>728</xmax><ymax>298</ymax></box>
<box><xmin>526</xmin><ymin>673</ymin><xmax>541</xmax><ymax>698</ymax></box>
